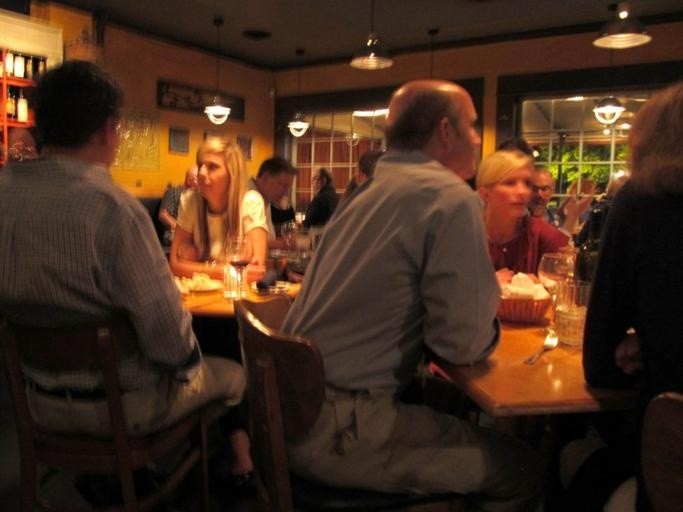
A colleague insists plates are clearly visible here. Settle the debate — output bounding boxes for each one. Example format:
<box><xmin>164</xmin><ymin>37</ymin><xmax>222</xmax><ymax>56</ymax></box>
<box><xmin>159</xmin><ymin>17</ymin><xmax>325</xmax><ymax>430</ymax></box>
<box><xmin>1</xmin><ymin>45</ymin><xmax>50</xmax><ymax>169</ymax></box>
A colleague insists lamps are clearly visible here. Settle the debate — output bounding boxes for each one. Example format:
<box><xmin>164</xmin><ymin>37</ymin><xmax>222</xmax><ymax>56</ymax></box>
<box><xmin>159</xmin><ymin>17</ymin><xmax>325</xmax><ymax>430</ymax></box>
<box><xmin>348</xmin><ymin>0</ymin><xmax>395</xmax><ymax>71</ymax></box>
<box><xmin>591</xmin><ymin>94</ymin><xmax>625</xmax><ymax>124</ymax></box>
<box><xmin>204</xmin><ymin>17</ymin><xmax>232</xmax><ymax>126</ymax></box>
<box><xmin>589</xmin><ymin>0</ymin><xmax>656</xmax><ymax>49</ymax></box>
<box><xmin>281</xmin><ymin>46</ymin><xmax>312</xmax><ymax>138</ymax></box>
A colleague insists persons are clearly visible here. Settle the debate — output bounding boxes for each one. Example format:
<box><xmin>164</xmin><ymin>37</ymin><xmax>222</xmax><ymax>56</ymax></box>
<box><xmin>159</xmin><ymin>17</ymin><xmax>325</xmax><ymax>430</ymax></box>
<box><xmin>160</xmin><ymin>166</ymin><xmax>198</xmax><ymax>248</ymax></box>
<box><xmin>559</xmin><ymin>84</ymin><xmax>681</xmax><ymax>510</ymax></box>
<box><xmin>0</xmin><ymin>57</ymin><xmax>248</xmax><ymax>493</ymax></box>
<box><xmin>270</xmin><ymin>195</ymin><xmax>296</xmax><ymax>237</ymax></box>
<box><xmin>526</xmin><ymin>169</ymin><xmax>628</xmax><ymax>283</ymax></box>
<box><xmin>248</xmin><ymin>156</ymin><xmax>299</xmax><ymax>283</ymax></box>
<box><xmin>426</xmin><ymin>148</ymin><xmax>573</xmax><ymax>444</ymax></box>
<box><xmin>249</xmin><ymin>78</ymin><xmax>550</xmax><ymax>511</ymax></box>
<box><xmin>474</xmin><ymin>150</ymin><xmax>573</xmax><ymax>284</ymax></box>
<box><xmin>299</xmin><ymin>168</ymin><xmax>340</xmax><ymax>229</ymax></box>
<box><xmin>170</xmin><ymin>133</ymin><xmax>280</xmax><ymax>491</ymax></box>
<box><xmin>354</xmin><ymin>151</ymin><xmax>384</xmax><ymax>187</ymax></box>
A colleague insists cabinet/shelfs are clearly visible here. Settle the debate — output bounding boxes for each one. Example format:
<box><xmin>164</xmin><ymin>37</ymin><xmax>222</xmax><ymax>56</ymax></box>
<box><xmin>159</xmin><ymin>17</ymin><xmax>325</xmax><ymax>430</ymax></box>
<box><xmin>1</xmin><ymin>45</ymin><xmax>50</xmax><ymax>169</ymax></box>
<box><xmin>16</xmin><ymin>88</ymin><xmax>28</xmax><ymax>122</ymax></box>
<box><xmin>6</xmin><ymin>89</ymin><xmax>17</xmax><ymax>119</ymax></box>
<box><xmin>4</xmin><ymin>50</ymin><xmax>46</xmax><ymax>79</ymax></box>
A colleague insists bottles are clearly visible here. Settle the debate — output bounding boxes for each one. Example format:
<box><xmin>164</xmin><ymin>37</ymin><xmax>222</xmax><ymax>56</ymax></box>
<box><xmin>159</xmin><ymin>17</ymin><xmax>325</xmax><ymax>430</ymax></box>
<box><xmin>359</xmin><ymin>150</ymin><xmax>381</xmax><ymax>179</ymax></box>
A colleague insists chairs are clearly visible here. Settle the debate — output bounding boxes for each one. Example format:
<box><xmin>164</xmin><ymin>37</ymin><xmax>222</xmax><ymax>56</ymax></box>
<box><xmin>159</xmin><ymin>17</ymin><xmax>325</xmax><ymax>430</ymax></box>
<box><xmin>1</xmin><ymin>294</ymin><xmax>466</xmax><ymax>511</ymax></box>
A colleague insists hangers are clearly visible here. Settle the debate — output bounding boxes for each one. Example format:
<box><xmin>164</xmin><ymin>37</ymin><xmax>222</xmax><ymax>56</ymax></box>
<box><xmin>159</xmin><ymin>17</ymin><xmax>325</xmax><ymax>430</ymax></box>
<box><xmin>523</xmin><ymin>330</ymin><xmax>558</xmax><ymax>365</ymax></box>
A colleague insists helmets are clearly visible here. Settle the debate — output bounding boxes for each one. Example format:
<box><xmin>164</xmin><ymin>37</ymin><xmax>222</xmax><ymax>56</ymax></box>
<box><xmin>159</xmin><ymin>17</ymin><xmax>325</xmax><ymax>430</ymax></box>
<box><xmin>190</xmin><ymin>279</ymin><xmax>223</xmax><ymax>292</ymax></box>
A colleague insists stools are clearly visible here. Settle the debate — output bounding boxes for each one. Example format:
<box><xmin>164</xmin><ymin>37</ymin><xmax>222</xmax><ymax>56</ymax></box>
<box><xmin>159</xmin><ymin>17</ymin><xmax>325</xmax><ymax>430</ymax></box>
<box><xmin>32</xmin><ymin>383</ymin><xmax>115</xmax><ymax>403</ymax></box>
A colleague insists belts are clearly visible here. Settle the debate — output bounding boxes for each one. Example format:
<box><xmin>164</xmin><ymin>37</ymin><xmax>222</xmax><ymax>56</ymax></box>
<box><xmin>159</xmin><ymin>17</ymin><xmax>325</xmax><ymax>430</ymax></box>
<box><xmin>226</xmin><ymin>234</ymin><xmax>254</xmax><ymax>302</ymax></box>
<box><xmin>538</xmin><ymin>253</ymin><xmax>572</xmax><ymax>334</ymax></box>
<box><xmin>559</xmin><ymin>247</ymin><xmax>580</xmax><ymax>282</ymax></box>
<box><xmin>293</xmin><ymin>230</ymin><xmax>310</xmax><ymax>272</ymax></box>
<box><xmin>281</xmin><ymin>222</ymin><xmax>296</xmax><ymax>242</ymax></box>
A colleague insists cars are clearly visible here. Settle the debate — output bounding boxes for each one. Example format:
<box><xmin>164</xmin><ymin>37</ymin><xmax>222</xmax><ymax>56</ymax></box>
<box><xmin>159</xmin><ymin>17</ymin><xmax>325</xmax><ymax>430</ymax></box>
<box><xmin>556</xmin><ymin>280</ymin><xmax>592</xmax><ymax>349</ymax></box>
<box><xmin>223</xmin><ymin>258</ymin><xmax>248</xmax><ymax>302</ymax></box>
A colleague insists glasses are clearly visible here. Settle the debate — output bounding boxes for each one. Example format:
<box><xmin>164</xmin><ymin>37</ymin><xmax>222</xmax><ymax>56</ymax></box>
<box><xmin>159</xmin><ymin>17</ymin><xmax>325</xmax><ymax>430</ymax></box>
<box><xmin>204</xmin><ymin>17</ymin><xmax>232</xmax><ymax>126</ymax></box>
<box><xmin>589</xmin><ymin>0</ymin><xmax>656</xmax><ymax>49</ymax></box>
<box><xmin>348</xmin><ymin>0</ymin><xmax>395</xmax><ymax>71</ymax></box>
<box><xmin>591</xmin><ymin>94</ymin><xmax>625</xmax><ymax>124</ymax></box>
<box><xmin>281</xmin><ymin>46</ymin><xmax>312</xmax><ymax>138</ymax></box>
